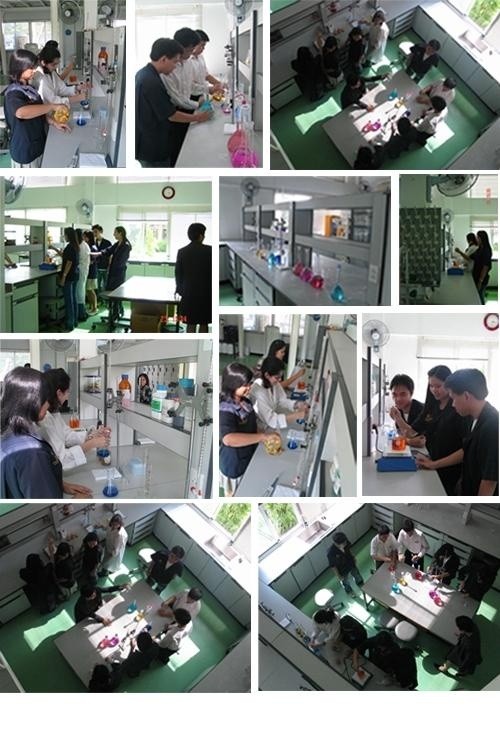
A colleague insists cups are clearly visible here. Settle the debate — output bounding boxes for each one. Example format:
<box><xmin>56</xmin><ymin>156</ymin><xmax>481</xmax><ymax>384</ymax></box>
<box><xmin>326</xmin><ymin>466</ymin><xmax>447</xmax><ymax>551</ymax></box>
<box><xmin>391</xmin><ymin>433</ymin><xmax>406</xmax><ymax>450</ymax></box>
<box><xmin>54</xmin><ymin>108</ymin><xmax>69</xmax><ymax>123</ymax></box>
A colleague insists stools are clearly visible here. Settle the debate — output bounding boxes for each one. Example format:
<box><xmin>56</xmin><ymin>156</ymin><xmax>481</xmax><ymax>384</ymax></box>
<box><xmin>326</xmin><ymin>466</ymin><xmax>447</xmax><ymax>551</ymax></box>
<box><xmin>379</xmin><ymin>609</ymin><xmax>404</xmax><ymax>630</ymax></box>
<box><xmin>313</xmin><ymin>588</ymin><xmax>344</xmax><ymax>611</ymax></box>
<box><xmin>394</xmin><ymin>619</ymin><xmax>418</xmax><ymax>642</ymax></box>
<box><xmin>397</xmin><ymin>40</ymin><xmax>416</xmax><ymax>64</ymax></box>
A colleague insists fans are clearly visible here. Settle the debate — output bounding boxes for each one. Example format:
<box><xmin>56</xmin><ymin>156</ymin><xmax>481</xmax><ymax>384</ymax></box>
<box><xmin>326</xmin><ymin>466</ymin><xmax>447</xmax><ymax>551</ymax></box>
<box><xmin>427</xmin><ymin>174</ymin><xmax>480</xmax><ymax>202</ymax></box>
<box><xmin>4</xmin><ymin>176</ymin><xmax>26</xmax><ymax>205</ymax></box>
<box><xmin>95</xmin><ymin>339</ymin><xmax>125</xmax><ymax>354</ymax></box>
<box><xmin>96</xmin><ymin>0</ymin><xmax>119</xmax><ymax>28</ymax></box>
<box><xmin>75</xmin><ymin>198</ymin><xmax>94</xmax><ymax>218</ymax></box>
<box><xmin>240</xmin><ymin>176</ymin><xmax>260</xmax><ymax>206</ymax></box>
<box><xmin>362</xmin><ymin>319</ymin><xmax>389</xmax><ymax>353</ymax></box>
<box><xmin>225</xmin><ymin>0</ymin><xmax>253</xmax><ymax>23</ymax></box>
<box><xmin>44</xmin><ymin>339</ymin><xmax>74</xmax><ymax>353</ymax></box>
<box><xmin>441</xmin><ymin>207</ymin><xmax>455</xmax><ymax>224</ymax></box>
<box><xmin>57</xmin><ymin>0</ymin><xmax>83</xmax><ymax>36</ymax></box>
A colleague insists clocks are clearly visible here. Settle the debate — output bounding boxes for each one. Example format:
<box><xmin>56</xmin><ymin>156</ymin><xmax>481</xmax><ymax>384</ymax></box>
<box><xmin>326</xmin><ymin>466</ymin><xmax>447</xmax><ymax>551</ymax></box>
<box><xmin>162</xmin><ymin>186</ymin><xmax>175</xmax><ymax>199</ymax></box>
<box><xmin>483</xmin><ymin>313</ymin><xmax>499</xmax><ymax>331</ymax></box>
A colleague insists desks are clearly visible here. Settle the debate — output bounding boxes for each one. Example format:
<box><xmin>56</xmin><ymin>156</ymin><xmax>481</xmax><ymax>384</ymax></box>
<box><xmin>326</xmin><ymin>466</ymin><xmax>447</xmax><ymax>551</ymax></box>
<box><xmin>108</xmin><ymin>276</ymin><xmax>181</xmax><ymax>333</ymax></box>
<box><xmin>55</xmin><ymin>581</ymin><xmax>176</xmax><ymax>688</ymax></box>
<box><xmin>361</xmin><ymin>561</ymin><xmax>481</xmax><ymax>645</ymax></box>
<box><xmin>322</xmin><ymin>70</ymin><xmax>433</xmax><ymax>168</ymax></box>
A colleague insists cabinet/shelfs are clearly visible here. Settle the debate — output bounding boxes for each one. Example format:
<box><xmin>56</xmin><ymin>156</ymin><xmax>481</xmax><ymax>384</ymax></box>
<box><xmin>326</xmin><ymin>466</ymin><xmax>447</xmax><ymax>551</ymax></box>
<box><xmin>5</xmin><ymin>272</ymin><xmax>85</xmax><ymax>332</ymax></box>
<box><xmin>259</xmin><ymin>504</ymin><xmax>499</xmax><ymax>603</ymax></box>
<box><xmin>0</xmin><ymin>587</ymin><xmax>32</xmax><ymax>625</ymax></box>
<box><xmin>270</xmin><ymin>78</ymin><xmax>303</xmax><ymax>111</ymax></box>
<box><xmin>224</xmin><ymin>247</ymin><xmax>241</xmax><ymax>290</ymax></box>
<box><xmin>127</xmin><ymin>510</ymin><xmax>251</xmax><ymax>629</ymax></box>
<box><xmin>411</xmin><ymin>0</ymin><xmax>500</xmax><ymax>115</ymax></box>
<box><xmin>239</xmin><ymin>260</ymin><xmax>289</xmax><ymax>306</ymax></box>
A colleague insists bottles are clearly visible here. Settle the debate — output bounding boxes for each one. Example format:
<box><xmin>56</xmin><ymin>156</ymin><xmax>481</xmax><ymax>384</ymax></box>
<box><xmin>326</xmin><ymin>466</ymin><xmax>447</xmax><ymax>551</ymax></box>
<box><xmin>63</xmin><ymin>128</ymin><xmax>72</xmax><ymax>137</ymax></box>
<box><xmin>199</xmin><ymin>93</ymin><xmax>214</xmax><ymax>117</ymax></box>
<box><xmin>103</xmin><ymin>469</ymin><xmax>119</xmax><ymax>497</ymax></box>
<box><xmin>395</xmin><ymin>96</ymin><xmax>407</xmax><ymax>108</ymax></box>
<box><xmin>287</xmin><ymin>430</ymin><xmax>297</xmax><ymax>449</ymax></box>
<box><xmin>294</xmin><ymin>620</ymin><xmax>320</xmax><ymax>656</ymax></box>
<box><xmin>328</xmin><ymin>264</ymin><xmax>345</xmax><ymax>302</ymax></box>
<box><xmin>117</xmin><ymin>374</ymin><xmax>131</xmax><ymax>404</ymax></box>
<box><xmin>265</xmin><ymin>432</ymin><xmax>281</xmax><ymax>453</ymax></box>
<box><xmin>216</xmin><ymin>76</ymin><xmax>259</xmax><ymax>169</ymax></box>
<box><xmin>389</xmin><ymin>87</ymin><xmax>398</xmax><ymax>99</ymax></box>
<box><xmin>69</xmin><ymin>53</ymin><xmax>91</xmax><ymax>109</ymax></box>
<box><xmin>127</xmin><ymin>600</ymin><xmax>153</xmax><ymax>631</ymax></box>
<box><xmin>356</xmin><ymin>669</ymin><xmax>365</xmax><ymax>680</ymax></box>
<box><xmin>76</xmin><ymin>106</ymin><xmax>86</xmax><ymax>125</ymax></box>
<box><xmin>429</xmin><ymin>590</ymin><xmax>441</xmax><ymax>605</ymax></box>
<box><xmin>374</xmin><ymin>119</ymin><xmax>381</xmax><ymax>131</ymax></box>
<box><xmin>151</xmin><ymin>383</ymin><xmax>193</xmax><ymax>434</ymax></box>
<box><xmin>310</xmin><ymin>254</ymin><xmax>325</xmax><ymax>288</ymax></box>
<box><xmin>95</xmin><ymin>424</ymin><xmax>110</xmax><ymax>467</ymax></box>
<box><xmin>111</xmin><ymin>634</ymin><xmax>119</xmax><ymax>646</ymax></box>
<box><xmin>267</xmin><ymin>238</ymin><xmax>288</xmax><ymax>266</ymax></box>
<box><xmin>69</xmin><ymin>408</ymin><xmax>81</xmax><ymax>429</ymax></box>
<box><xmin>97</xmin><ymin>45</ymin><xmax>118</xmax><ymax>81</ymax></box>
<box><xmin>387</xmin><ymin>429</ymin><xmax>394</xmax><ymax>445</ymax></box>
<box><xmin>145</xmin><ymin>448</ymin><xmax>153</xmax><ymax>497</ymax></box>
<box><xmin>94</xmin><ymin>111</ymin><xmax>109</xmax><ymax>154</ymax></box>
<box><xmin>296</xmin><ymin>418</ymin><xmax>310</xmax><ymax>449</ymax></box>
<box><xmin>367</xmin><ymin>121</ymin><xmax>371</xmax><ymax>132</ymax></box>
<box><xmin>107</xmin><ymin>76</ymin><xmax>112</xmax><ymax>111</ymax></box>
<box><xmin>298</xmin><ymin>375</ymin><xmax>305</xmax><ymax>389</ymax></box>
<box><xmin>293</xmin><ymin>247</ymin><xmax>310</xmax><ymax>282</ymax></box>
<box><xmin>101</xmin><ymin>636</ymin><xmax>109</xmax><ymax>648</ymax></box>
<box><xmin>388</xmin><ymin>562</ymin><xmax>422</xmax><ymax>594</ymax></box>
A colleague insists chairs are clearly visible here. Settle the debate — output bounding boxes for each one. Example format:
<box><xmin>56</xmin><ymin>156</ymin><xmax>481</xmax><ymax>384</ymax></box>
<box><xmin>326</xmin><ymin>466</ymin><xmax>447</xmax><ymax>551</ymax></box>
<box><xmin>339</xmin><ymin>614</ymin><xmax>367</xmax><ymax>655</ymax></box>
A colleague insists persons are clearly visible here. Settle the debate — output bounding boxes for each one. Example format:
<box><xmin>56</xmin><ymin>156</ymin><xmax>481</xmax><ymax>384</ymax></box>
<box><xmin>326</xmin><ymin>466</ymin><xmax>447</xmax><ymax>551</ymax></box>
<box><xmin>27</xmin><ymin>47</ymin><xmax>95</xmax><ymax>106</ymax></box>
<box><xmin>0</xmin><ymin>362</ymin><xmax>112</xmax><ymax>499</ymax></box>
<box><xmin>290</xmin><ymin>5</ymin><xmax>458</xmax><ymax>169</ymax></box>
<box><xmin>188</xmin><ymin>28</ymin><xmax>222</xmax><ymax>85</ymax></box>
<box><xmin>136</xmin><ymin>373</ymin><xmax>152</xmax><ymax>405</ymax></box>
<box><xmin>454</xmin><ymin>230</ymin><xmax>493</xmax><ymax>305</ymax></box>
<box><xmin>175</xmin><ymin>223</ymin><xmax>212</xmax><ymax>333</ymax></box>
<box><xmin>219</xmin><ymin>361</ymin><xmax>284</xmax><ymax>497</ymax></box>
<box><xmin>304</xmin><ymin>517</ymin><xmax>497</xmax><ymax>689</ymax></box>
<box><xmin>135</xmin><ymin>37</ymin><xmax>214</xmax><ymax>168</ymax></box>
<box><xmin>463</xmin><ymin>232</ymin><xmax>479</xmax><ymax>272</ymax></box>
<box><xmin>244</xmin><ymin>353</ymin><xmax>309</xmax><ymax>431</ymax></box>
<box><xmin>44</xmin><ymin>40</ymin><xmax>75</xmax><ymax>81</ymax></box>
<box><xmin>269</xmin><ymin>339</ymin><xmax>306</xmax><ymax>389</ymax></box>
<box><xmin>20</xmin><ymin>513</ymin><xmax>204</xmax><ymax>692</ymax></box>
<box><xmin>388</xmin><ymin>365</ymin><xmax>499</xmax><ymax>496</ymax></box>
<box><xmin>47</xmin><ymin>223</ymin><xmax>132</xmax><ymax>330</ymax></box>
<box><xmin>159</xmin><ymin>26</ymin><xmax>222</xmax><ymax>152</ymax></box>
<box><xmin>3</xmin><ymin>48</ymin><xmax>72</xmax><ymax>168</ymax></box>
